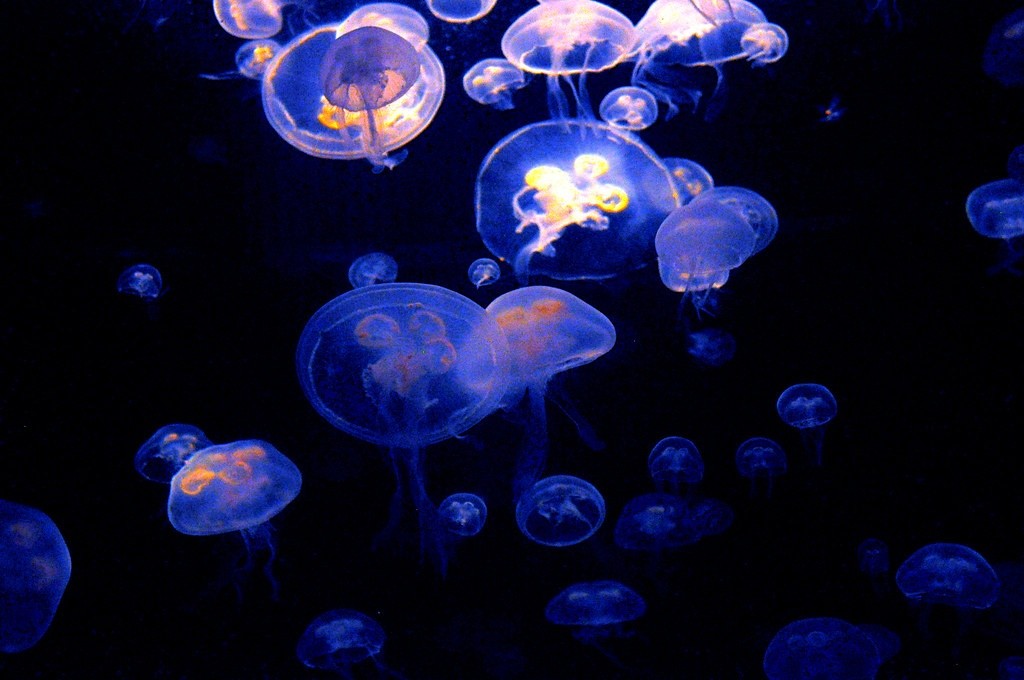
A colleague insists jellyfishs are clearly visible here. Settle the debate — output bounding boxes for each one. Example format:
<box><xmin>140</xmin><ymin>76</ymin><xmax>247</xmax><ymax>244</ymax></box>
<box><xmin>0</xmin><ymin>0</ymin><xmax>1024</xmax><ymax>680</ymax></box>
